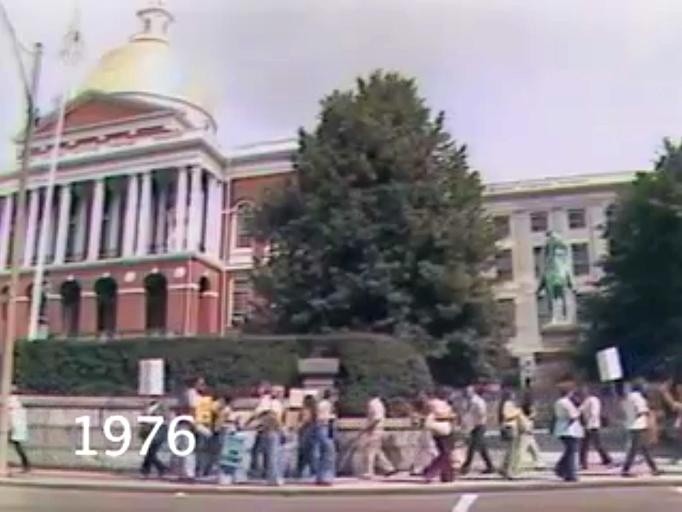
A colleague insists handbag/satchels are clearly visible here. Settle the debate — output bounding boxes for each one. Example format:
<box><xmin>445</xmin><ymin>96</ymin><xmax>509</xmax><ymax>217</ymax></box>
<box><xmin>424</xmin><ymin>411</ymin><xmax>451</xmax><ymax>436</ymax></box>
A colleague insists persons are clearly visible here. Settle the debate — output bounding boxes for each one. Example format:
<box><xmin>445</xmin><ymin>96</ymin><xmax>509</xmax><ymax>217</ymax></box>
<box><xmin>554</xmin><ymin>381</ymin><xmax>579</xmax><ymax>483</ymax></box>
<box><xmin>187</xmin><ymin>377</ymin><xmax>286</xmax><ymax>484</ymax></box>
<box><xmin>312</xmin><ymin>389</ymin><xmax>339</xmax><ymax>485</ymax></box>
<box><xmin>621</xmin><ymin>377</ymin><xmax>664</xmax><ymax>477</ymax></box>
<box><xmin>138</xmin><ymin>402</ymin><xmax>169</xmax><ymax>477</ymax></box>
<box><xmin>656</xmin><ymin>381</ymin><xmax>682</xmax><ymax>427</ymax></box>
<box><xmin>579</xmin><ymin>386</ymin><xmax>613</xmax><ymax>469</ymax></box>
<box><xmin>458</xmin><ymin>386</ymin><xmax>495</xmax><ymax>476</ymax></box>
<box><xmin>500</xmin><ymin>377</ymin><xmax>546</xmax><ymax>478</ymax></box>
<box><xmin>294</xmin><ymin>394</ymin><xmax>315</xmax><ymax>479</ymax></box>
<box><xmin>7</xmin><ymin>395</ymin><xmax>29</xmax><ymax>474</ymax></box>
<box><xmin>421</xmin><ymin>385</ymin><xmax>456</xmax><ymax>482</ymax></box>
<box><xmin>358</xmin><ymin>390</ymin><xmax>397</xmax><ymax>478</ymax></box>
